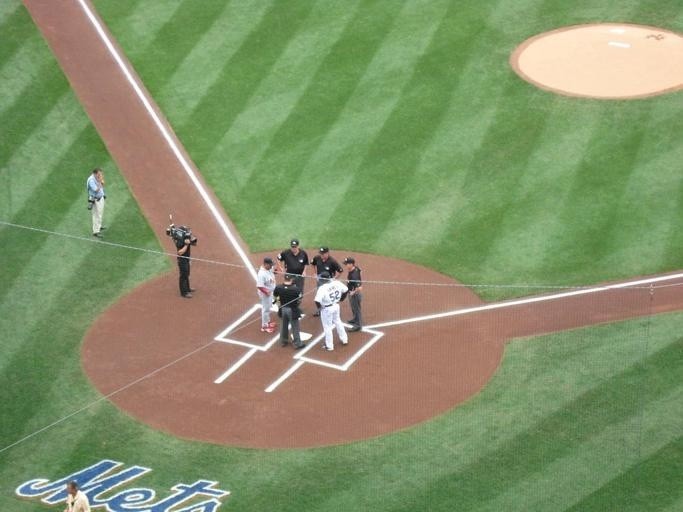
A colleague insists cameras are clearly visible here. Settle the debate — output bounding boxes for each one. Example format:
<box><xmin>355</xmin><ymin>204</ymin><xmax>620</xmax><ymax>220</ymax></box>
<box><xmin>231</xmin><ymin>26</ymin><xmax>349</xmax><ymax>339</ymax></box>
<box><xmin>87</xmin><ymin>199</ymin><xmax>95</xmax><ymax>210</ymax></box>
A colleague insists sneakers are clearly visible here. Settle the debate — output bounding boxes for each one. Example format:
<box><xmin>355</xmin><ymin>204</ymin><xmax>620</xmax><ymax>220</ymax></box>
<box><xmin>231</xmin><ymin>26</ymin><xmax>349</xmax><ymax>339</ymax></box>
<box><xmin>261</xmin><ymin>311</ymin><xmax>361</xmax><ymax>351</ymax></box>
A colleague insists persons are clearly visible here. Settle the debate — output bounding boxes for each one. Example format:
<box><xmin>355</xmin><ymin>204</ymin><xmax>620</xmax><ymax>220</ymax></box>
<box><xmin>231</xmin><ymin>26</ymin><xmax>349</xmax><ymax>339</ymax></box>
<box><xmin>86</xmin><ymin>168</ymin><xmax>106</xmax><ymax>238</ymax></box>
<box><xmin>275</xmin><ymin>239</ymin><xmax>309</xmax><ymax>295</ymax></box>
<box><xmin>272</xmin><ymin>274</ymin><xmax>305</xmax><ymax>349</ymax></box>
<box><xmin>176</xmin><ymin>225</ymin><xmax>195</xmax><ymax>298</ymax></box>
<box><xmin>313</xmin><ymin>271</ymin><xmax>349</xmax><ymax>351</ymax></box>
<box><xmin>343</xmin><ymin>257</ymin><xmax>363</xmax><ymax>333</ymax></box>
<box><xmin>255</xmin><ymin>256</ymin><xmax>277</xmax><ymax>333</ymax></box>
<box><xmin>63</xmin><ymin>481</ymin><xmax>90</xmax><ymax>512</ymax></box>
<box><xmin>310</xmin><ymin>246</ymin><xmax>343</xmax><ymax>317</ymax></box>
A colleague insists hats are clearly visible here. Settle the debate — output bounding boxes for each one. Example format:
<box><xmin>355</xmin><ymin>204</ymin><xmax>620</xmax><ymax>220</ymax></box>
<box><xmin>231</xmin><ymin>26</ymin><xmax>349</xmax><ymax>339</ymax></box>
<box><xmin>319</xmin><ymin>246</ymin><xmax>328</xmax><ymax>254</ymax></box>
<box><xmin>342</xmin><ymin>256</ymin><xmax>354</xmax><ymax>264</ymax></box>
<box><xmin>291</xmin><ymin>238</ymin><xmax>298</xmax><ymax>247</ymax></box>
<box><xmin>264</xmin><ymin>256</ymin><xmax>276</xmax><ymax>266</ymax></box>
